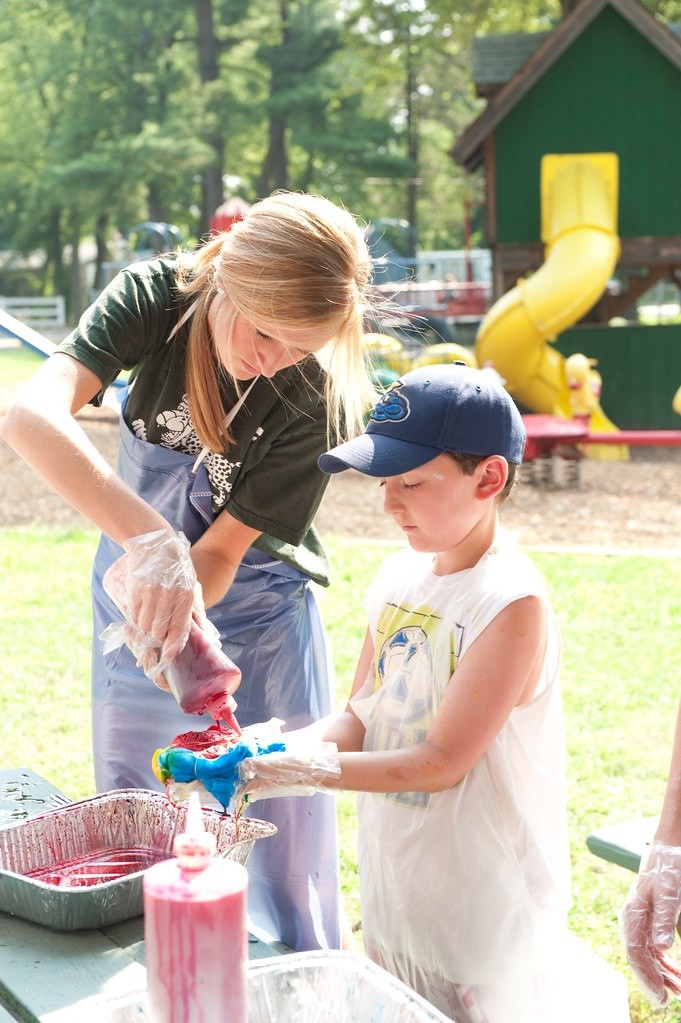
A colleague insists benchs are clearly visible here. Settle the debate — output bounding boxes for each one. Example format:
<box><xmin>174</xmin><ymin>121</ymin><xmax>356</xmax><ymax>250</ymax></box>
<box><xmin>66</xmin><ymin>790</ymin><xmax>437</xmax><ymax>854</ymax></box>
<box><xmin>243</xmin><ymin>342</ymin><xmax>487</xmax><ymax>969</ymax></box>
<box><xmin>0</xmin><ymin>766</ymin><xmax>284</xmax><ymax>1023</ymax></box>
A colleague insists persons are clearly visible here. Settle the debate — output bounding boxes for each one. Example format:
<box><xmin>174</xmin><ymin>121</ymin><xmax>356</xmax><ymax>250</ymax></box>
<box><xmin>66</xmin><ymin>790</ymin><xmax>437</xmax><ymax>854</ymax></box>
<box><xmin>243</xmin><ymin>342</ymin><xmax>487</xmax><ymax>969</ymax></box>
<box><xmin>159</xmin><ymin>365</ymin><xmax>566</xmax><ymax>1022</ymax></box>
<box><xmin>625</xmin><ymin>690</ymin><xmax>681</xmax><ymax>1003</ymax></box>
<box><xmin>0</xmin><ymin>190</ymin><xmax>368</xmax><ymax>951</ymax></box>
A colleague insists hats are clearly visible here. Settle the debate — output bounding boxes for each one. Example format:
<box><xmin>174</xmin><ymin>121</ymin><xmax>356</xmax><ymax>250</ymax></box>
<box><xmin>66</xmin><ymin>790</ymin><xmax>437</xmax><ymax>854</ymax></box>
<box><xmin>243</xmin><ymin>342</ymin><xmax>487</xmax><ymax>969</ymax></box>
<box><xmin>318</xmin><ymin>359</ymin><xmax>526</xmax><ymax>475</ymax></box>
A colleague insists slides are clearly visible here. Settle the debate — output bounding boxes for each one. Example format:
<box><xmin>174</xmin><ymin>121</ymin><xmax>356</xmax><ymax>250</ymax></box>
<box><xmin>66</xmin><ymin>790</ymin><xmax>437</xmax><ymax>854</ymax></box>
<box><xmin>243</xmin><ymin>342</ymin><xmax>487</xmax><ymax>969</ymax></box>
<box><xmin>473</xmin><ymin>207</ymin><xmax>637</xmax><ymax>463</ymax></box>
